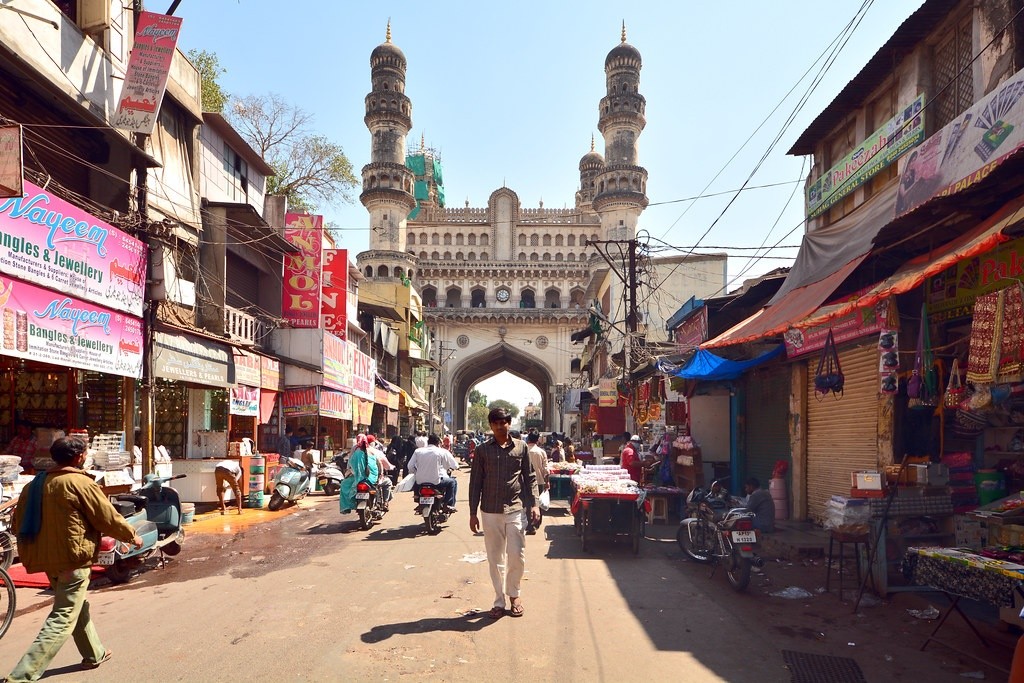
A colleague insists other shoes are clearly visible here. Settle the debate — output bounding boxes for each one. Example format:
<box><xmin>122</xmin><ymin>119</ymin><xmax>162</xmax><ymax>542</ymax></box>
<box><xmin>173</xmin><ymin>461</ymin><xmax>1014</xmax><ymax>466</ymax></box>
<box><xmin>442</xmin><ymin>506</ymin><xmax>457</xmax><ymax>513</ymax></box>
<box><xmin>526</xmin><ymin>530</ymin><xmax>536</xmax><ymax>535</ymax></box>
<box><xmin>414</xmin><ymin>495</ymin><xmax>419</xmax><ymax>502</ymax></box>
<box><xmin>383</xmin><ymin>501</ymin><xmax>389</xmax><ymax>512</ymax></box>
<box><xmin>341</xmin><ymin>509</ymin><xmax>351</xmax><ymax>514</ymax></box>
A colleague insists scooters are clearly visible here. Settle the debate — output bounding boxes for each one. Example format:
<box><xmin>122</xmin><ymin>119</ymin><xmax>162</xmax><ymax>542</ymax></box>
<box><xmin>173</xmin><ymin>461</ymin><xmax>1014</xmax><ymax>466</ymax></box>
<box><xmin>91</xmin><ymin>474</ymin><xmax>187</xmax><ymax>585</ymax></box>
<box><xmin>314</xmin><ymin>450</ymin><xmax>351</xmax><ymax>496</ymax></box>
<box><xmin>269</xmin><ymin>455</ymin><xmax>312</xmax><ymax>510</ymax></box>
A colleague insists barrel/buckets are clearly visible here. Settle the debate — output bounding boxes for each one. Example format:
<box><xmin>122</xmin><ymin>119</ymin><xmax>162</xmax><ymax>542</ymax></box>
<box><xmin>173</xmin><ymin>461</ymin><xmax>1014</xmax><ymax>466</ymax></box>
<box><xmin>768</xmin><ymin>477</ymin><xmax>788</xmax><ymax>499</ymax></box>
<box><xmin>773</xmin><ymin>500</ymin><xmax>789</xmax><ymax>520</ymax></box>
<box><xmin>249</xmin><ymin>491</ymin><xmax>264</xmax><ymax>507</ymax></box>
<box><xmin>181</xmin><ymin>502</ymin><xmax>195</xmax><ymax>525</ymax></box>
<box><xmin>249</xmin><ymin>457</ymin><xmax>265</xmax><ymax>474</ymax></box>
<box><xmin>249</xmin><ymin>474</ymin><xmax>264</xmax><ymax>491</ymax></box>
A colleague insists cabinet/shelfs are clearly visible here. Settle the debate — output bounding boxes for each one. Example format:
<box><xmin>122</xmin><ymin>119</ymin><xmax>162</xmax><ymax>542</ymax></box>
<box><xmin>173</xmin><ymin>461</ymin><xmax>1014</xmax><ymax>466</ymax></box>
<box><xmin>549</xmin><ymin>453</ymin><xmax>648</xmax><ymax>554</ymax></box>
<box><xmin>139</xmin><ymin>384</ymin><xmax>185</xmax><ymax>458</ymax></box>
<box><xmin>34</xmin><ymin>467</ymin><xmax>132</xmax><ymax>495</ymax></box>
<box><xmin>859</xmin><ymin>513</ymin><xmax>955</xmax><ymax>597</ymax></box>
<box><xmin>982</xmin><ymin>425</ymin><xmax>1024</xmax><ymax>455</ymax></box>
<box><xmin>203</xmin><ymin>454</ymin><xmax>278</xmax><ymax>497</ymax></box>
<box><xmin>0</xmin><ymin>367</ymin><xmax>124</xmax><ymax>441</ymax></box>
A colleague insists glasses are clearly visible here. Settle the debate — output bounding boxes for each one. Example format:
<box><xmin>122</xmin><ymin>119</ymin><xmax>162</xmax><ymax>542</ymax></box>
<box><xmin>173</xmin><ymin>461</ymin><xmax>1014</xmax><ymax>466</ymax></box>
<box><xmin>637</xmin><ymin>441</ymin><xmax>641</xmax><ymax>443</ymax></box>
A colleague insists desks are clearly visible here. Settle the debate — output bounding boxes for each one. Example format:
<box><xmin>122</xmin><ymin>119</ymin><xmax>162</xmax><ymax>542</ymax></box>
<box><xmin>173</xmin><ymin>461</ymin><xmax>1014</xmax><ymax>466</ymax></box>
<box><xmin>899</xmin><ymin>546</ymin><xmax>1024</xmax><ymax>674</ymax></box>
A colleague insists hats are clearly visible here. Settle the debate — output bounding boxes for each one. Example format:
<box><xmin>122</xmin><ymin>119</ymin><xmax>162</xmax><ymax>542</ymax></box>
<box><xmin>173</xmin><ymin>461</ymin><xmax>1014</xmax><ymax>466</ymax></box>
<box><xmin>630</xmin><ymin>435</ymin><xmax>641</xmax><ymax>440</ymax></box>
<box><xmin>367</xmin><ymin>435</ymin><xmax>375</xmax><ymax>443</ymax></box>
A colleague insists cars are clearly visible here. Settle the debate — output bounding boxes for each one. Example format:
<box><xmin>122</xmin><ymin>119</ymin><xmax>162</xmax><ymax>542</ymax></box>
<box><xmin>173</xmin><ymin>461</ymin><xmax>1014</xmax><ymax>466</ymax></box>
<box><xmin>538</xmin><ymin>431</ymin><xmax>563</xmax><ymax>444</ymax></box>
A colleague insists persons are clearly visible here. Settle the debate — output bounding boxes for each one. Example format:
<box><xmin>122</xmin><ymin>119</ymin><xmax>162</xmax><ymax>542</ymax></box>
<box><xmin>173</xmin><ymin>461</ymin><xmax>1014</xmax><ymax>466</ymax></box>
<box><xmin>339</xmin><ymin>428</ymin><xmax>492</xmax><ymax>516</ymax></box>
<box><xmin>277</xmin><ymin>426</ymin><xmax>334</xmax><ymax>480</ymax></box>
<box><xmin>509</xmin><ymin>427</ymin><xmax>662</xmax><ymax>536</ymax></box>
<box><xmin>707</xmin><ymin>477</ymin><xmax>776</xmax><ymax>533</ymax></box>
<box><xmin>468</xmin><ymin>408</ymin><xmax>540</xmax><ymax>619</ymax></box>
<box><xmin>214</xmin><ymin>459</ymin><xmax>245</xmax><ymax>515</ymax></box>
<box><xmin>0</xmin><ymin>437</ymin><xmax>143</xmax><ymax>682</ymax></box>
<box><xmin>3</xmin><ymin>419</ymin><xmax>38</xmax><ymax>474</ymax></box>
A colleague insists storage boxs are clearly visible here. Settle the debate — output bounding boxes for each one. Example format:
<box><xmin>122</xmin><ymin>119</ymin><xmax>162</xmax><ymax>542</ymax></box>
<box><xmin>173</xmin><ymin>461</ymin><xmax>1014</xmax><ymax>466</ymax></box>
<box><xmin>856</xmin><ymin>469</ymin><xmax>886</xmax><ymax>489</ymax></box>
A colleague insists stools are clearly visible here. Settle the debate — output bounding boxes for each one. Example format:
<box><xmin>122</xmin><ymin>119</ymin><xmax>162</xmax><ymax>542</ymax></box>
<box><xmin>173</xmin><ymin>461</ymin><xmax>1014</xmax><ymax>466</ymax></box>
<box><xmin>826</xmin><ymin>531</ymin><xmax>875</xmax><ymax>603</ymax></box>
<box><xmin>649</xmin><ymin>494</ymin><xmax>669</xmax><ymax>525</ymax></box>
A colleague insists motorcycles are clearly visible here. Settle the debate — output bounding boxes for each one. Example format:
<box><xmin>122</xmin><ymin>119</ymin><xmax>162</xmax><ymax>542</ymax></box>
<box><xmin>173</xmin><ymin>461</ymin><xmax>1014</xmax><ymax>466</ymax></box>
<box><xmin>354</xmin><ymin>467</ymin><xmax>397</xmax><ymax>531</ymax></box>
<box><xmin>414</xmin><ymin>468</ymin><xmax>458</xmax><ymax>535</ymax></box>
<box><xmin>455</xmin><ymin>430</ymin><xmax>475</xmax><ymax>443</ymax></box>
<box><xmin>675</xmin><ymin>475</ymin><xmax>765</xmax><ymax>590</ymax></box>
<box><xmin>483</xmin><ymin>433</ymin><xmax>494</xmax><ymax>440</ymax></box>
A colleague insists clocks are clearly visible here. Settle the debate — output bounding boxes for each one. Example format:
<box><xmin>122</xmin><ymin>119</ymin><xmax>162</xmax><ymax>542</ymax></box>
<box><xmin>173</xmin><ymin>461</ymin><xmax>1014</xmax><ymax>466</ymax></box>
<box><xmin>497</xmin><ymin>289</ymin><xmax>509</xmax><ymax>301</ymax></box>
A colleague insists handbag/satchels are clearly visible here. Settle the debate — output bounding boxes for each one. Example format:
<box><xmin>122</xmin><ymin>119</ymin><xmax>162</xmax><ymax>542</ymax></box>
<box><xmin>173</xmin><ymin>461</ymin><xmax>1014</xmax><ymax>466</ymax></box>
<box><xmin>539</xmin><ymin>489</ymin><xmax>550</xmax><ymax>511</ymax></box>
<box><xmin>907</xmin><ymin>373</ymin><xmax>920</xmax><ymax>398</ymax></box>
<box><xmin>393</xmin><ymin>473</ymin><xmax>414</xmax><ymax>493</ymax></box>
<box><xmin>944</xmin><ymin>359</ymin><xmax>964</xmax><ymax>410</ymax></box>
<box><xmin>813</xmin><ymin>371</ymin><xmax>844</xmax><ymax>396</ymax></box>
<box><xmin>924</xmin><ymin>367</ymin><xmax>938</xmax><ymax>392</ymax></box>
<box><xmin>387</xmin><ymin>447</ymin><xmax>397</xmax><ymax>464</ymax></box>
<box><xmin>954</xmin><ymin>398</ymin><xmax>987</xmax><ymax>438</ymax></box>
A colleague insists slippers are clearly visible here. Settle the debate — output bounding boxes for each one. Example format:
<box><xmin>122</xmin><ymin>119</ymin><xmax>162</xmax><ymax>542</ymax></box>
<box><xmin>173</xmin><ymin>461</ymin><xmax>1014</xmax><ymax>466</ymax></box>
<box><xmin>488</xmin><ymin>607</ymin><xmax>505</xmax><ymax>617</ymax></box>
<box><xmin>80</xmin><ymin>651</ymin><xmax>112</xmax><ymax>666</ymax></box>
<box><xmin>510</xmin><ymin>596</ymin><xmax>524</xmax><ymax>616</ymax></box>
